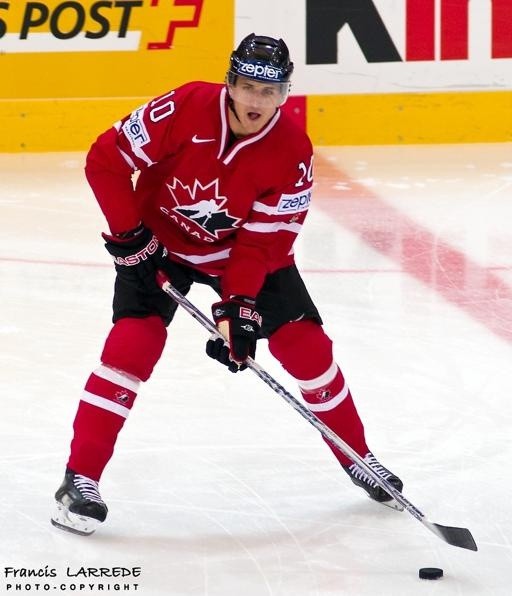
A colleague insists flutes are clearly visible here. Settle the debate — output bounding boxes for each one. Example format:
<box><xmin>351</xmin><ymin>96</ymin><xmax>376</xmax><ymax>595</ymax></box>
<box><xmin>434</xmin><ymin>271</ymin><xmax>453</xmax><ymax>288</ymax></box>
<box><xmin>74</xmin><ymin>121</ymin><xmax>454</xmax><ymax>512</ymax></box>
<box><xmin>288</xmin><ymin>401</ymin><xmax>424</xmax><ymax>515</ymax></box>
<box><xmin>156</xmin><ymin>271</ymin><xmax>478</xmax><ymax>552</ymax></box>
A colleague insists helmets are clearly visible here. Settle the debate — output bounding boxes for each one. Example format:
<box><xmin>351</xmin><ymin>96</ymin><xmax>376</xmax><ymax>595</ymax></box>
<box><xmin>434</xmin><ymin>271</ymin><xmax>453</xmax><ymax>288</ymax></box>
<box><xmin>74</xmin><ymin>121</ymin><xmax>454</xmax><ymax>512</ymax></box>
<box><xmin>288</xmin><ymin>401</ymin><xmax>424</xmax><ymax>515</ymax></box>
<box><xmin>224</xmin><ymin>31</ymin><xmax>295</xmax><ymax>90</ymax></box>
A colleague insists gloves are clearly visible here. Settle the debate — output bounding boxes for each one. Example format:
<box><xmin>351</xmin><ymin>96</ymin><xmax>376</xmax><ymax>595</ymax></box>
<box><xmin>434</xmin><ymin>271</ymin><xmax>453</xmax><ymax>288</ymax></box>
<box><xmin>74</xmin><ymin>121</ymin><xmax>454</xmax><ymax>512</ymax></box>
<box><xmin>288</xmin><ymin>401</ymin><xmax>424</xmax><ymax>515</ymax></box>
<box><xmin>100</xmin><ymin>227</ymin><xmax>173</xmax><ymax>300</ymax></box>
<box><xmin>200</xmin><ymin>292</ymin><xmax>257</xmax><ymax>375</ymax></box>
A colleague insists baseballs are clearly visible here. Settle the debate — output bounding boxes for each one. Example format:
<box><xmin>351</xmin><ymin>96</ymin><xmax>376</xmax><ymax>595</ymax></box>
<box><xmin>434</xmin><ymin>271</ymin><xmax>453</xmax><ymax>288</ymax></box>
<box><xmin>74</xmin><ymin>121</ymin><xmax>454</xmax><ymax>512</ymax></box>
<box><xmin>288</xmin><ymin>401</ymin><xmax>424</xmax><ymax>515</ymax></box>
<box><xmin>419</xmin><ymin>568</ymin><xmax>443</xmax><ymax>580</ymax></box>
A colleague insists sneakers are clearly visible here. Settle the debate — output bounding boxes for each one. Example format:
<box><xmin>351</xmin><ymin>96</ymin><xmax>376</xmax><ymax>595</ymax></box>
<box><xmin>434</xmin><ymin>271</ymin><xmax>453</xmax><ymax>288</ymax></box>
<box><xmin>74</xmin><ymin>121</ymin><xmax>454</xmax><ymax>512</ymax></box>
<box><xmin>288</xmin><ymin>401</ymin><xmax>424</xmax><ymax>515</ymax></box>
<box><xmin>341</xmin><ymin>451</ymin><xmax>400</xmax><ymax>506</ymax></box>
<box><xmin>55</xmin><ymin>462</ymin><xmax>108</xmax><ymax>522</ymax></box>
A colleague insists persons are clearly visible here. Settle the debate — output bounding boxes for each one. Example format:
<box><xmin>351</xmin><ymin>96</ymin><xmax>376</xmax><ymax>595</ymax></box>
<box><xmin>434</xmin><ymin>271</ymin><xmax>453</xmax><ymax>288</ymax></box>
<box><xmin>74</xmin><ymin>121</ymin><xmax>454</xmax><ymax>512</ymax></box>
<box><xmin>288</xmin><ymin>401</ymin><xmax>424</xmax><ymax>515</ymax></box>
<box><xmin>50</xmin><ymin>33</ymin><xmax>403</xmax><ymax>524</ymax></box>
